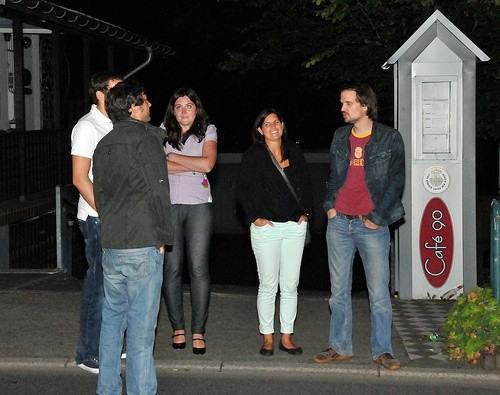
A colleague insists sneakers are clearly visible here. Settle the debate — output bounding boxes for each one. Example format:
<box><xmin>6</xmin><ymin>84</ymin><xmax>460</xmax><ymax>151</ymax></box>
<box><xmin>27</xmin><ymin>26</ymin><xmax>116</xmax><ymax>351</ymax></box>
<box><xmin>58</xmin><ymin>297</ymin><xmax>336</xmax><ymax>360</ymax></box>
<box><xmin>373</xmin><ymin>352</ymin><xmax>402</xmax><ymax>370</ymax></box>
<box><xmin>313</xmin><ymin>346</ymin><xmax>353</xmax><ymax>362</ymax></box>
<box><xmin>121</xmin><ymin>347</ymin><xmax>127</xmax><ymax>359</ymax></box>
<box><xmin>77</xmin><ymin>357</ymin><xmax>100</xmax><ymax>374</ymax></box>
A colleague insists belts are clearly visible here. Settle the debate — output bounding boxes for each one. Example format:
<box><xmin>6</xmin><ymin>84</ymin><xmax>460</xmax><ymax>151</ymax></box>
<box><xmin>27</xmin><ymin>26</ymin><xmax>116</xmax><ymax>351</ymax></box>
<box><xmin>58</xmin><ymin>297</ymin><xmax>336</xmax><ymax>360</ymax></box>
<box><xmin>337</xmin><ymin>213</ymin><xmax>369</xmax><ymax>220</ymax></box>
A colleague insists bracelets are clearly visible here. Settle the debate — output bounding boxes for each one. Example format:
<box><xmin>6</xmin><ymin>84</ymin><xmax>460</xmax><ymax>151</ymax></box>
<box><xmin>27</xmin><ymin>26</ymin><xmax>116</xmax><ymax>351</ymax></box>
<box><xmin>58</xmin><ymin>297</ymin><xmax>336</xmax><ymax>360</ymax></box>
<box><xmin>166</xmin><ymin>152</ymin><xmax>170</xmax><ymax>160</ymax></box>
<box><xmin>254</xmin><ymin>217</ymin><xmax>260</xmax><ymax>223</ymax></box>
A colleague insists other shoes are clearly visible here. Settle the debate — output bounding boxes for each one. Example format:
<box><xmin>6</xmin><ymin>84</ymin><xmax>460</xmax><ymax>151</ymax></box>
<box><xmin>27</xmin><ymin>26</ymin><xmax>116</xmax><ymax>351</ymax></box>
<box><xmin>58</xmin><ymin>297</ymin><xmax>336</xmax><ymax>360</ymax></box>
<box><xmin>192</xmin><ymin>338</ymin><xmax>206</xmax><ymax>354</ymax></box>
<box><xmin>279</xmin><ymin>341</ymin><xmax>303</xmax><ymax>355</ymax></box>
<box><xmin>260</xmin><ymin>346</ymin><xmax>274</xmax><ymax>356</ymax></box>
<box><xmin>171</xmin><ymin>330</ymin><xmax>186</xmax><ymax>349</ymax></box>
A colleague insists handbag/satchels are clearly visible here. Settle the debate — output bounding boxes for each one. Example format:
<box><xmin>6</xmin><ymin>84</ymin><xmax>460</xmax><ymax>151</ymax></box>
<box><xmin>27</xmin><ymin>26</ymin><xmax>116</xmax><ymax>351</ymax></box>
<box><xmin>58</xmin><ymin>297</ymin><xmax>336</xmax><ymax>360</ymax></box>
<box><xmin>304</xmin><ymin>220</ymin><xmax>313</xmax><ymax>246</ymax></box>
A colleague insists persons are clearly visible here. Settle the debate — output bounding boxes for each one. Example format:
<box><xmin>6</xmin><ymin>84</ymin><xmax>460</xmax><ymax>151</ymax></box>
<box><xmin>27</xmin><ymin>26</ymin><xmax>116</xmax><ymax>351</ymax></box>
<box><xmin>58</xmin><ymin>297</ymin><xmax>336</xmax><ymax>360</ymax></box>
<box><xmin>235</xmin><ymin>106</ymin><xmax>322</xmax><ymax>358</ymax></box>
<box><xmin>69</xmin><ymin>72</ymin><xmax>127</xmax><ymax>375</ymax></box>
<box><xmin>313</xmin><ymin>82</ymin><xmax>406</xmax><ymax>372</ymax></box>
<box><xmin>159</xmin><ymin>86</ymin><xmax>218</xmax><ymax>354</ymax></box>
<box><xmin>93</xmin><ymin>79</ymin><xmax>176</xmax><ymax>395</ymax></box>
